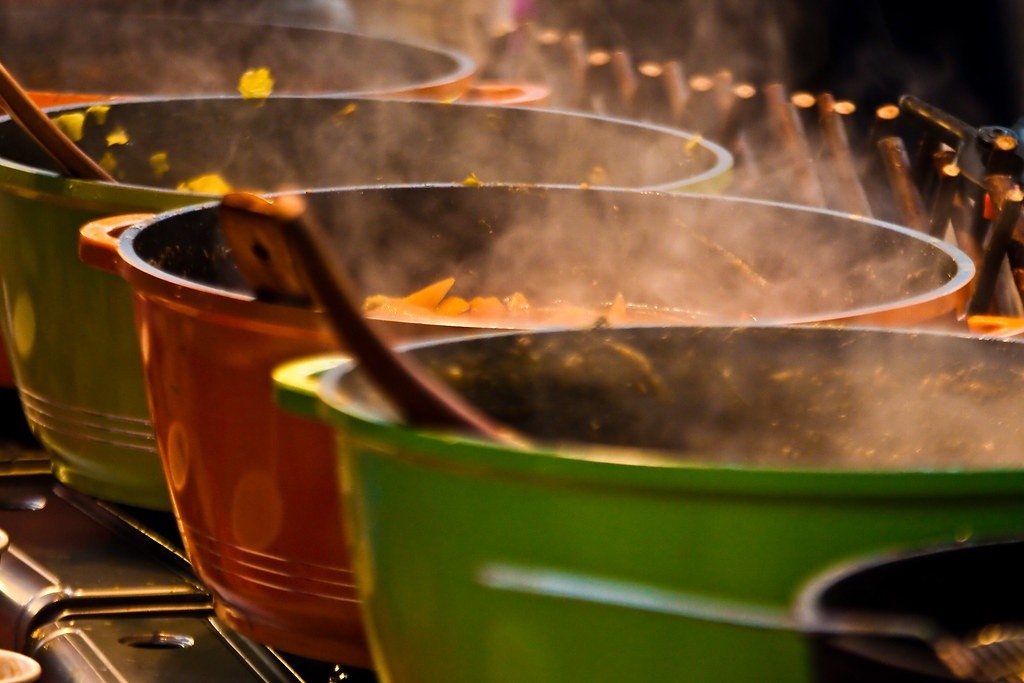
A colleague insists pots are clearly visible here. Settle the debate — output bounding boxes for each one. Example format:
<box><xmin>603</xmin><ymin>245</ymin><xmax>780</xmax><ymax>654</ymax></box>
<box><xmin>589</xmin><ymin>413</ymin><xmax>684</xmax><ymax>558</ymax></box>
<box><xmin>0</xmin><ymin>16</ymin><xmax>1024</xmax><ymax>683</ymax></box>
